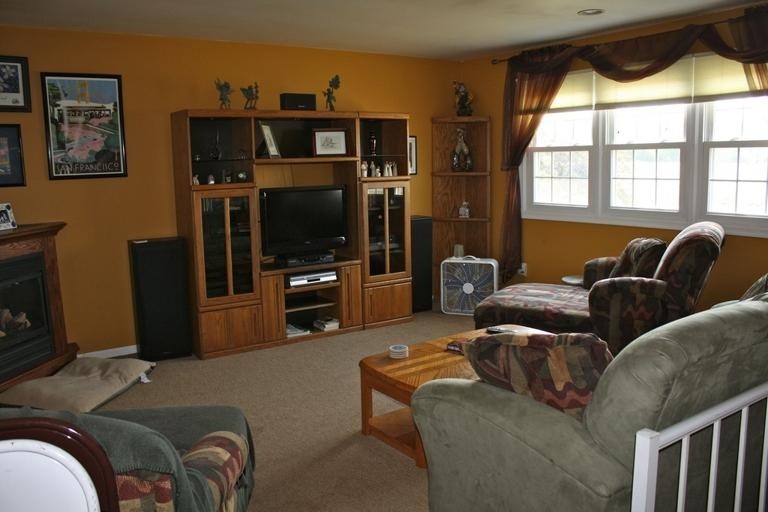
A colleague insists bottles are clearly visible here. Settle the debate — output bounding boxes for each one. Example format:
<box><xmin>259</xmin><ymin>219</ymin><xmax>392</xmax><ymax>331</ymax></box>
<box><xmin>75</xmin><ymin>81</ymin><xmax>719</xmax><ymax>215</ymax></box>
<box><xmin>361</xmin><ymin>160</ymin><xmax>398</xmax><ymax>177</ymax></box>
<box><xmin>368</xmin><ymin>127</ymin><xmax>378</xmax><ymax>157</ymax></box>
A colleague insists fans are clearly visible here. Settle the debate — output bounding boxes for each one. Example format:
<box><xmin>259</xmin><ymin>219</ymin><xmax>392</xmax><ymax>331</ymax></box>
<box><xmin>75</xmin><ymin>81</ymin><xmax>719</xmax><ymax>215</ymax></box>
<box><xmin>439</xmin><ymin>254</ymin><xmax>499</xmax><ymax>317</ymax></box>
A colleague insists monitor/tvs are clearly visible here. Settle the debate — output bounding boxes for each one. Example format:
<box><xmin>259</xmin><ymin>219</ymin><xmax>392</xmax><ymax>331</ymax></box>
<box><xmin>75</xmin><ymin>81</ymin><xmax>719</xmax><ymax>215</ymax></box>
<box><xmin>260</xmin><ymin>185</ymin><xmax>348</xmax><ymax>256</ymax></box>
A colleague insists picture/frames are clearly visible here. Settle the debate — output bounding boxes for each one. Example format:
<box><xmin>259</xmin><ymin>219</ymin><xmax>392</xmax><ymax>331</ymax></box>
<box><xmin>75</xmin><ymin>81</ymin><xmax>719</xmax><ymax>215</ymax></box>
<box><xmin>312</xmin><ymin>127</ymin><xmax>351</xmax><ymax>157</ymax></box>
<box><xmin>0</xmin><ymin>54</ymin><xmax>128</xmax><ymax>188</ymax></box>
<box><xmin>258</xmin><ymin>120</ymin><xmax>282</xmax><ymax>159</ymax></box>
<box><xmin>410</xmin><ymin>135</ymin><xmax>417</xmax><ymax>174</ymax></box>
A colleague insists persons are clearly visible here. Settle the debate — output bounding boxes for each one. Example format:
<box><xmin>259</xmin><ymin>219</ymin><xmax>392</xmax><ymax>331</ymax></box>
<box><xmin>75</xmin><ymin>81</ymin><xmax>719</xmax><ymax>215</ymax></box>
<box><xmin>322</xmin><ymin>87</ymin><xmax>336</xmax><ymax>108</ymax></box>
<box><xmin>244</xmin><ymin>85</ymin><xmax>254</xmax><ymax>109</ymax></box>
<box><xmin>218</xmin><ymin>81</ymin><xmax>231</xmax><ymax>109</ymax></box>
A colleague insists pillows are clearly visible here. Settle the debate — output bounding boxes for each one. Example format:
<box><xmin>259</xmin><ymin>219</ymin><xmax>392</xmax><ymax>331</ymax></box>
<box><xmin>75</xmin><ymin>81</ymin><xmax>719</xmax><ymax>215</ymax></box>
<box><xmin>0</xmin><ymin>357</ymin><xmax>157</xmax><ymax>415</ymax></box>
<box><xmin>460</xmin><ymin>331</ymin><xmax>614</xmax><ymax>423</ymax></box>
<box><xmin>608</xmin><ymin>236</ymin><xmax>668</xmax><ymax>278</ymax></box>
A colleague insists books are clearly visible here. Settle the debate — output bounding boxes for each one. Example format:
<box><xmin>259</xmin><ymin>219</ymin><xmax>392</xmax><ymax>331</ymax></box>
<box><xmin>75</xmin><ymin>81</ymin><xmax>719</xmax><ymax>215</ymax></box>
<box><xmin>286</xmin><ymin>316</ymin><xmax>339</xmax><ymax>337</ymax></box>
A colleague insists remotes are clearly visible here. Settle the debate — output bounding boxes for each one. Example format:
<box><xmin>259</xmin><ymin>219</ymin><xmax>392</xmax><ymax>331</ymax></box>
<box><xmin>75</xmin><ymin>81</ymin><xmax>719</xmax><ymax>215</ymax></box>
<box><xmin>486</xmin><ymin>327</ymin><xmax>510</xmax><ymax>333</ymax></box>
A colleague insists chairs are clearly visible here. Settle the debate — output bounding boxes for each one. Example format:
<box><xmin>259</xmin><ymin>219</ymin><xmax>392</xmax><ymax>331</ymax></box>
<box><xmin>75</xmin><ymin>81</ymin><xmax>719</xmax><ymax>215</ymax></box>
<box><xmin>473</xmin><ymin>220</ymin><xmax>726</xmax><ymax>357</ymax></box>
<box><xmin>1</xmin><ymin>405</ymin><xmax>256</xmax><ymax>512</ymax></box>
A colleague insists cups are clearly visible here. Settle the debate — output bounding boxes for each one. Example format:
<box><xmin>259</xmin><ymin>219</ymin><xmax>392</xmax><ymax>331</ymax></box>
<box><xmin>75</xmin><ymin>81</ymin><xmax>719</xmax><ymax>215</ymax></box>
<box><xmin>453</xmin><ymin>244</ymin><xmax>465</xmax><ymax>256</ymax></box>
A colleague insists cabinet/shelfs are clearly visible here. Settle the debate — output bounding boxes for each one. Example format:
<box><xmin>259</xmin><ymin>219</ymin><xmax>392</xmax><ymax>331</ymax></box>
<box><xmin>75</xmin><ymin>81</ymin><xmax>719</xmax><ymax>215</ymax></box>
<box><xmin>0</xmin><ymin>223</ymin><xmax>81</xmax><ymax>392</ymax></box>
<box><xmin>169</xmin><ymin>109</ymin><xmax>416</xmax><ymax>361</ymax></box>
<box><xmin>430</xmin><ymin>114</ymin><xmax>493</xmax><ymax>313</ymax></box>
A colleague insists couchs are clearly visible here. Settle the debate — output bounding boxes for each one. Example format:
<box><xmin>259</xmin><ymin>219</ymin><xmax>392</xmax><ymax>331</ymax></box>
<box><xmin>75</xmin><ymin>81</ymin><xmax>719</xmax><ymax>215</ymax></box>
<box><xmin>408</xmin><ymin>258</ymin><xmax>767</xmax><ymax>512</ymax></box>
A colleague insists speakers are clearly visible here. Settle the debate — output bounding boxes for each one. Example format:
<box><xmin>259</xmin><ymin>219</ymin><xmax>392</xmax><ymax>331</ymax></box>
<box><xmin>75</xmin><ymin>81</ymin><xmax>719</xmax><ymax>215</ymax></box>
<box><xmin>281</xmin><ymin>92</ymin><xmax>316</xmax><ymax>110</ymax></box>
<box><xmin>411</xmin><ymin>214</ymin><xmax>433</xmax><ymax>313</ymax></box>
<box><xmin>128</xmin><ymin>237</ymin><xmax>193</xmax><ymax>361</ymax></box>
